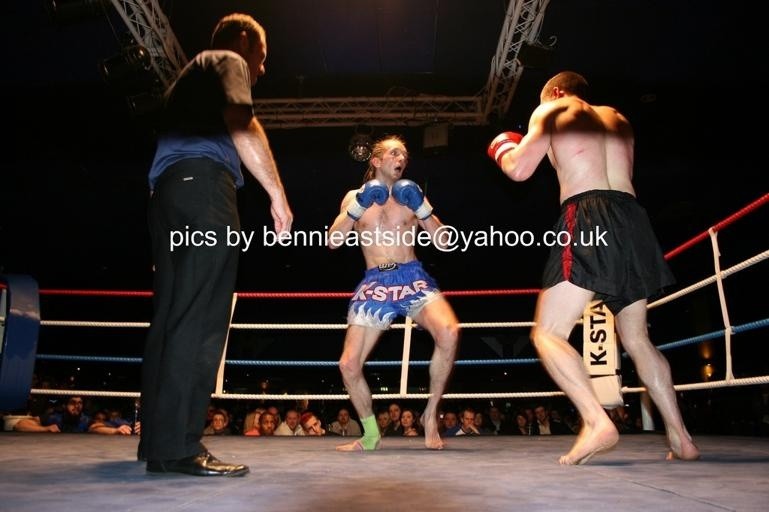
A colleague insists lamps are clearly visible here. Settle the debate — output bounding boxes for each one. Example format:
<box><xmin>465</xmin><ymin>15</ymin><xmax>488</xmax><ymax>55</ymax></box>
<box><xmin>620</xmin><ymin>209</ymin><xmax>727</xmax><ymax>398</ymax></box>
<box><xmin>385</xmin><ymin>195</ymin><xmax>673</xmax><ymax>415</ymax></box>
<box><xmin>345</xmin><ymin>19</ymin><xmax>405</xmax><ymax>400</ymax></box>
<box><xmin>102</xmin><ymin>35</ymin><xmax>153</xmax><ymax>82</ymax></box>
<box><xmin>351</xmin><ymin>122</ymin><xmax>372</xmax><ymax>162</ymax></box>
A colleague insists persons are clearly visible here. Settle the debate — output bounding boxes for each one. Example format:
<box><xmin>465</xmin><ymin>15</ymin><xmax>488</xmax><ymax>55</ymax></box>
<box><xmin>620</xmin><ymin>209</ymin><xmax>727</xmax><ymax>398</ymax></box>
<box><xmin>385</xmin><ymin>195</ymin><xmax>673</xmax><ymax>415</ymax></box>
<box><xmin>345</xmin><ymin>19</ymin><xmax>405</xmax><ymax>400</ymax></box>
<box><xmin>486</xmin><ymin>70</ymin><xmax>701</xmax><ymax>467</ymax></box>
<box><xmin>136</xmin><ymin>14</ymin><xmax>294</xmax><ymax>475</ymax></box>
<box><xmin>328</xmin><ymin>135</ymin><xmax>460</xmax><ymax>452</ymax></box>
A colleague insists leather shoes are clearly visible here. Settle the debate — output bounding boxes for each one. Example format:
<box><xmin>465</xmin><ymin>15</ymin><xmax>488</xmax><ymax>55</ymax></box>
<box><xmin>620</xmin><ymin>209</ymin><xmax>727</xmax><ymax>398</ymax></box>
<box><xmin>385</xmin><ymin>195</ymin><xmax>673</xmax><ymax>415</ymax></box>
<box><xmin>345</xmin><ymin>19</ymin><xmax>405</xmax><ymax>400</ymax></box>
<box><xmin>145</xmin><ymin>448</ymin><xmax>249</xmax><ymax>477</ymax></box>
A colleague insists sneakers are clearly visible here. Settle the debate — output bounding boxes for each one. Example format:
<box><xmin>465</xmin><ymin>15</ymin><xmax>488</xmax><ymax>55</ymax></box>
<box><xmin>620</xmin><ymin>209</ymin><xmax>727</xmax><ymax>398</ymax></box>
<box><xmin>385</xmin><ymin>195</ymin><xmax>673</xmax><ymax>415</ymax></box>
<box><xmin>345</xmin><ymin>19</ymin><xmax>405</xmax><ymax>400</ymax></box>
<box><xmin>136</xmin><ymin>439</ymin><xmax>152</xmax><ymax>462</ymax></box>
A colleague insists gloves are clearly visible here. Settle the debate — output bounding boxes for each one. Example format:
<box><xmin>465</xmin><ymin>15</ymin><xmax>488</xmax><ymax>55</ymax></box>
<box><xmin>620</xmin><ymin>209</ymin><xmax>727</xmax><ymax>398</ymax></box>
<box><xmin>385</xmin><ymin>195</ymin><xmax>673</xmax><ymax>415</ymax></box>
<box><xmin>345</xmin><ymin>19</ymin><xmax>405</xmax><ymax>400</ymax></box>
<box><xmin>347</xmin><ymin>179</ymin><xmax>389</xmax><ymax>222</ymax></box>
<box><xmin>488</xmin><ymin>131</ymin><xmax>524</xmax><ymax>168</ymax></box>
<box><xmin>391</xmin><ymin>179</ymin><xmax>434</xmax><ymax>220</ymax></box>
